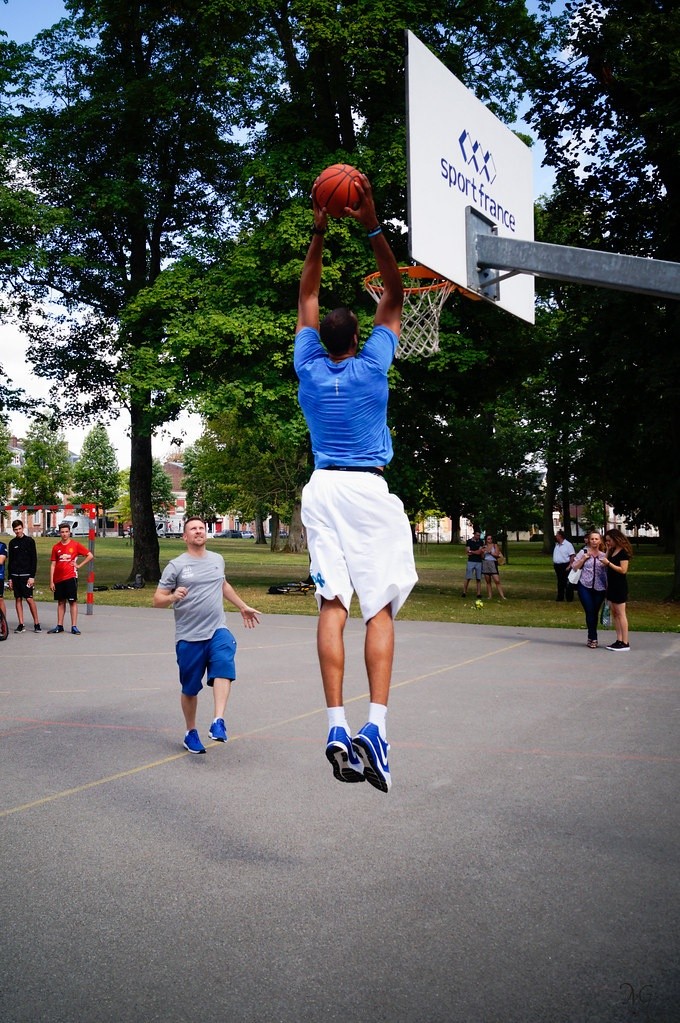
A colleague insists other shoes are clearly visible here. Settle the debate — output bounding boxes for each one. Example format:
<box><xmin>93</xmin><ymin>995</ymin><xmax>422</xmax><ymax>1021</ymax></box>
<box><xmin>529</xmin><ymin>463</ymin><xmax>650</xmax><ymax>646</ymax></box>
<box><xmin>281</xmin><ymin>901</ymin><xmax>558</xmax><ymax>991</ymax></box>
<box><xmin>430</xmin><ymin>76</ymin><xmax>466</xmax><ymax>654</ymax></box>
<box><xmin>461</xmin><ymin>594</ymin><xmax>466</xmax><ymax>598</ymax></box>
<box><xmin>590</xmin><ymin>639</ymin><xmax>598</xmax><ymax>648</ymax></box>
<box><xmin>477</xmin><ymin>595</ymin><xmax>481</xmax><ymax>599</ymax></box>
<box><xmin>587</xmin><ymin>638</ymin><xmax>592</xmax><ymax>647</ymax></box>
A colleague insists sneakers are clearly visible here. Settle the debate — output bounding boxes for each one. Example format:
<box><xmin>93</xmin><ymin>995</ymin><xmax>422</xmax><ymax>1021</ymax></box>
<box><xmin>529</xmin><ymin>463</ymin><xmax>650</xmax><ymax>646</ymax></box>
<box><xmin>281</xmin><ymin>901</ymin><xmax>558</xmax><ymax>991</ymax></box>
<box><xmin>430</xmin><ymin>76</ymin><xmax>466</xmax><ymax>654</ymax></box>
<box><xmin>209</xmin><ymin>718</ymin><xmax>227</xmax><ymax>743</ymax></box>
<box><xmin>14</xmin><ymin>624</ymin><xmax>25</xmax><ymax>634</ymax></box>
<box><xmin>52</xmin><ymin>625</ymin><xmax>64</xmax><ymax>633</ymax></box>
<box><xmin>606</xmin><ymin>640</ymin><xmax>622</xmax><ymax>649</ymax></box>
<box><xmin>71</xmin><ymin>626</ymin><xmax>81</xmax><ymax>634</ymax></box>
<box><xmin>352</xmin><ymin>722</ymin><xmax>392</xmax><ymax>793</ymax></box>
<box><xmin>183</xmin><ymin>729</ymin><xmax>206</xmax><ymax>754</ymax></box>
<box><xmin>325</xmin><ymin>726</ymin><xmax>365</xmax><ymax>783</ymax></box>
<box><xmin>34</xmin><ymin>623</ymin><xmax>42</xmax><ymax>633</ymax></box>
<box><xmin>611</xmin><ymin>641</ymin><xmax>630</xmax><ymax>651</ymax></box>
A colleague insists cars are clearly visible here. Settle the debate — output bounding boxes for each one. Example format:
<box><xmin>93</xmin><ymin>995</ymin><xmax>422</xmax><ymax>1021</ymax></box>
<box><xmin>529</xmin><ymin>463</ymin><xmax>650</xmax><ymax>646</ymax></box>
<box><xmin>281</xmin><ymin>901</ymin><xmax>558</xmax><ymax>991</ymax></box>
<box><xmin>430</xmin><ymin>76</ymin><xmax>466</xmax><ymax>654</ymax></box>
<box><xmin>240</xmin><ymin>531</ymin><xmax>289</xmax><ymax>540</ymax></box>
<box><xmin>212</xmin><ymin>530</ymin><xmax>242</xmax><ymax>539</ymax></box>
<box><xmin>41</xmin><ymin>528</ymin><xmax>57</xmax><ymax>537</ymax></box>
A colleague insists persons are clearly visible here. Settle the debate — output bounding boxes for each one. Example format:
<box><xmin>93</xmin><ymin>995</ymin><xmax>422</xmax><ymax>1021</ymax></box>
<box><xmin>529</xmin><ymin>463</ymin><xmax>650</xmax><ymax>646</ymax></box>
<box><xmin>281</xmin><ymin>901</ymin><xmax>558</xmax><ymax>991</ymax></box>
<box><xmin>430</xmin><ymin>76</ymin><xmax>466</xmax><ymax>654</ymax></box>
<box><xmin>153</xmin><ymin>516</ymin><xmax>262</xmax><ymax>754</ymax></box>
<box><xmin>572</xmin><ymin>530</ymin><xmax>608</xmax><ymax>648</ymax></box>
<box><xmin>0</xmin><ymin>542</ymin><xmax>9</xmax><ymax>618</ymax></box>
<box><xmin>293</xmin><ymin>174</ymin><xmax>419</xmax><ymax>793</ymax></box>
<box><xmin>47</xmin><ymin>523</ymin><xmax>94</xmax><ymax>635</ymax></box>
<box><xmin>552</xmin><ymin>531</ymin><xmax>576</xmax><ymax>602</ymax></box>
<box><xmin>461</xmin><ymin>529</ymin><xmax>485</xmax><ymax>599</ymax></box>
<box><xmin>7</xmin><ymin>519</ymin><xmax>42</xmax><ymax>633</ymax></box>
<box><xmin>598</xmin><ymin>528</ymin><xmax>633</xmax><ymax>651</ymax></box>
<box><xmin>481</xmin><ymin>534</ymin><xmax>509</xmax><ymax>600</ymax></box>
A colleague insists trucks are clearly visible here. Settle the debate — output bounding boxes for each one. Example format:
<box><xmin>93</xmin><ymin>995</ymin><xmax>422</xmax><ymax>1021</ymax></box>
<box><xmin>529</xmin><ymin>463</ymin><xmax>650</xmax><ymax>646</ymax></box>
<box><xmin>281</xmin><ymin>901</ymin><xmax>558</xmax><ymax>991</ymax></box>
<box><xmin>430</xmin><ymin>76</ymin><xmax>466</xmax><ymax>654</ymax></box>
<box><xmin>156</xmin><ymin>518</ymin><xmax>183</xmax><ymax>538</ymax></box>
<box><xmin>55</xmin><ymin>516</ymin><xmax>90</xmax><ymax>537</ymax></box>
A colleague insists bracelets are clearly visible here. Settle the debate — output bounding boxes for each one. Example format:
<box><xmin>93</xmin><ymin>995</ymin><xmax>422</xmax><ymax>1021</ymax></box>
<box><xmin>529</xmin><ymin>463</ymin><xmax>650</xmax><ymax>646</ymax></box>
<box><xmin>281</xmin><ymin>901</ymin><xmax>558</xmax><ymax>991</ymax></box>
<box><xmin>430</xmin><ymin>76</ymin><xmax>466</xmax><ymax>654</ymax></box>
<box><xmin>607</xmin><ymin>561</ymin><xmax>611</xmax><ymax>566</ymax></box>
<box><xmin>366</xmin><ymin>225</ymin><xmax>381</xmax><ymax>234</ymax></box>
<box><xmin>312</xmin><ymin>227</ymin><xmax>327</xmax><ymax>234</ymax></box>
<box><xmin>468</xmin><ymin>551</ymin><xmax>471</xmax><ymax>553</ymax></box>
<box><xmin>368</xmin><ymin>228</ymin><xmax>382</xmax><ymax>238</ymax></box>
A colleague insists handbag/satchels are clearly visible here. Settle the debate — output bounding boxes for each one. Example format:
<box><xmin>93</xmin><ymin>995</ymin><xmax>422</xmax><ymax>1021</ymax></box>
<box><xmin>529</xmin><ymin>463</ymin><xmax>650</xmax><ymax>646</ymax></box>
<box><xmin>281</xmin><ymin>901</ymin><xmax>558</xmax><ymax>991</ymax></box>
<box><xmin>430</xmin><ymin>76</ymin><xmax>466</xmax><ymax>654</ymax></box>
<box><xmin>568</xmin><ymin>569</ymin><xmax>582</xmax><ymax>584</ymax></box>
<box><xmin>497</xmin><ymin>552</ymin><xmax>504</xmax><ymax>565</ymax></box>
<box><xmin>601</xmin><ymin>599</ymin><xmax>611</xmax><ymax>626</ymax></box>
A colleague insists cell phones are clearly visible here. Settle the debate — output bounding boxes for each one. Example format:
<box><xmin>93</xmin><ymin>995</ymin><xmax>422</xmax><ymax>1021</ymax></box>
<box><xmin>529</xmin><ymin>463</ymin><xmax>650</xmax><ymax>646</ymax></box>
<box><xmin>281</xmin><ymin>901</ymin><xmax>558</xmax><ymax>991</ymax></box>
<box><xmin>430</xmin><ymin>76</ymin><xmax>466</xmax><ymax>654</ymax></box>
<box><xmin>26</xmin><ymin>584</ymin><xmax>31</xmax><ymax>588</ymax></box>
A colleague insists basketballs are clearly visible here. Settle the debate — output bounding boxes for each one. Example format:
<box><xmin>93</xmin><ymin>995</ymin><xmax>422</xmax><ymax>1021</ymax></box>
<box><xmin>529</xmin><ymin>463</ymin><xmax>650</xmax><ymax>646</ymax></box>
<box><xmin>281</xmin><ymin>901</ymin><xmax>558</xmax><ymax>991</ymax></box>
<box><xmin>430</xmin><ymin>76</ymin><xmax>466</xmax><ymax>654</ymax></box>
<box><xmin>314</xmin><ymin>163</ymin><xmax>364</xmax><ymax>219</ymax></box>
